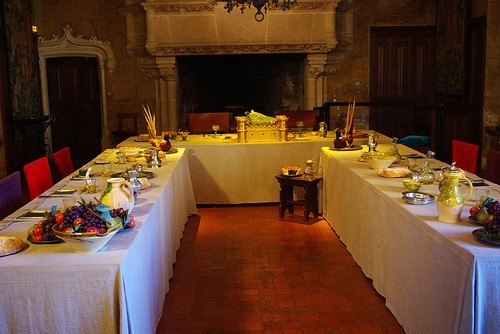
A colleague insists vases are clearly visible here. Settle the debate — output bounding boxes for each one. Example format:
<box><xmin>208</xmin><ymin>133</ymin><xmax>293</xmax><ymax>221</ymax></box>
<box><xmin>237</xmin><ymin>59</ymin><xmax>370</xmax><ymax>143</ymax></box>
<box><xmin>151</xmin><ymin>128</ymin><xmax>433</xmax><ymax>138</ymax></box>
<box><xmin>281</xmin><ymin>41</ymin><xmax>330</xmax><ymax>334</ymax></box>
<box><xmin>100</xmin><ymin>178</ymin><xmax>135</xmax><ymax>215</ymax></box>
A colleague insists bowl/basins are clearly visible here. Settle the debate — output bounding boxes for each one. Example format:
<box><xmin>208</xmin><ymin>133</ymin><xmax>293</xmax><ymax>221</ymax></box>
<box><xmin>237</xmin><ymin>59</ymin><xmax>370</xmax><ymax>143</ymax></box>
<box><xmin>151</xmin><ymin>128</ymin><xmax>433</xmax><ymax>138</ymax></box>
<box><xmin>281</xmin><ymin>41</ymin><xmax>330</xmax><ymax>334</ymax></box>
<box><xmin>53</xmin><ymin>219</ymin><xmax>122</xmax><ymax>252</ymax></box>
<box><xmin>362</xmin><ymin>152</ymin><xmax>397</xmax><ymax>170</ymax></box>
<box><xmin>401</xmin><ymin>179</ymin><xmax>435</xmax><ymax>206</ymax></box>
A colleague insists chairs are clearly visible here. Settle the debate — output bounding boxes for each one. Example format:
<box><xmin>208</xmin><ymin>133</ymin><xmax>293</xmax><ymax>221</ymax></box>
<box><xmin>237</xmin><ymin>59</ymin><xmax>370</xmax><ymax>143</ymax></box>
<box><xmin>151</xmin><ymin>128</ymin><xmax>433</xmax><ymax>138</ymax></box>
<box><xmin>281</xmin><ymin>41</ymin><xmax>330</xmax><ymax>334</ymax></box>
<box><xmin>189</xmin><ymin>112</ymin><xmax>230</xmax><ymax>135</ymax></box>
<box><xmin>0</xmin><ymin>147</ymin><xmax>73</xmax><ymax>221</ymax></box>
<box><xmin>398</xmin><ymin>135</ymin><xmax>500</xmax><ymax>185</ymax></box>
<box><xmin>273</xmin><ymin>110</ymin><xmax>317</xmax><ymax>128</ymax></box>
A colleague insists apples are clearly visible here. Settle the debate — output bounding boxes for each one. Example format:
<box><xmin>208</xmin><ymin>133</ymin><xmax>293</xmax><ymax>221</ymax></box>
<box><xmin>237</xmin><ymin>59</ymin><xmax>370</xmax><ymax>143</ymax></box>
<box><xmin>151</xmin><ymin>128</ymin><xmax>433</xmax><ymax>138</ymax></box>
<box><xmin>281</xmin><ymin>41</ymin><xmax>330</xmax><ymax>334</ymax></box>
<box><xmin>469</xmin><ymin>206</ymin><xmax>480</xmax><ymax>218</ymax></box>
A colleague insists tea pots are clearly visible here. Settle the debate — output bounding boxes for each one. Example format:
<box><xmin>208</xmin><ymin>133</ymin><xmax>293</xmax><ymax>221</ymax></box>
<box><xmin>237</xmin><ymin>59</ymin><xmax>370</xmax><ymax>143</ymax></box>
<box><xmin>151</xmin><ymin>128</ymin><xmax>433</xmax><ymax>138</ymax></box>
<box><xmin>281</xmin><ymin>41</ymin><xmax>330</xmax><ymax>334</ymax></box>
<box><xmin>318</xmin><ymin>122</ymin><xmax>328</xmax><ymax>137</ymax></box>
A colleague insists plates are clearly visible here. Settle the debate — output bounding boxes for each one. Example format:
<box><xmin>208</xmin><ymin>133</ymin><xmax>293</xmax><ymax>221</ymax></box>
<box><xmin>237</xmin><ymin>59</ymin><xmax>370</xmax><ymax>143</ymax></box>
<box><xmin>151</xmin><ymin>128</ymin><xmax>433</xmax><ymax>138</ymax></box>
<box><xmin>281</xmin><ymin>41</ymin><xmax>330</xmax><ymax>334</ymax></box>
<box><xmin>377</xmin><ymin>170</ymin><xmax>410</xmax><ymax>178</ymax></box>
<box><xmin>280</xmin><ymin>172</ymin><xmax>302</xmax><ymax>177</ymax></box>
<box><xmin>0</xmin><ymin>234</ymin><xmax>25</xmax><ymax>258</ymax></box>
<box><xmin>468</xmin><ymin>213</ymin><xmax>500</xmax><ymax>246</ymax></box>
<box><xmin>26</xmin><ymin>236</ymin><xmax>62</xmax><ymax>244</ymax></box>
<box><xmin>352</xmin><ymin>132</ymin><xmax>367</xmax><ymax>138</ymax></box>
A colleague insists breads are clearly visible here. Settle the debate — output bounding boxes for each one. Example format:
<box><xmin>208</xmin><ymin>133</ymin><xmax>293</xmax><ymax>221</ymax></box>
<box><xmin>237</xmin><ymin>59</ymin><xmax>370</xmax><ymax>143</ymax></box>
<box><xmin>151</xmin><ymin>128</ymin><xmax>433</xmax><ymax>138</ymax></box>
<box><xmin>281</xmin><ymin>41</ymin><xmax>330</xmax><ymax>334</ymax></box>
<box><xmin>0</xmin><ymin>236</ymin><xmax>25</xmax><ymax>255</ymax></box>
<box><xmin>382</xmin><ymin>169</ymin><xmax>411</xmax><ymax>177</ymax></box>
<box><xmin>126</xmin><ymin>156</ymin><xmax>136</xmax><ymax>162</ymax></box>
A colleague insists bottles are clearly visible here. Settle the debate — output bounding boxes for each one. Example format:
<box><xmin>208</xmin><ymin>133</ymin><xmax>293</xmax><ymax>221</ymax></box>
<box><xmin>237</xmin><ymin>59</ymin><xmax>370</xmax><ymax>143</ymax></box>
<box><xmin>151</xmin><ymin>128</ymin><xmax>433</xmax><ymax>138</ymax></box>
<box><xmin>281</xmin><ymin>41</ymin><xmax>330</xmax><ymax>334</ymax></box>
<box><xmin>212</xmin><ymin>125</ymin><xmax>220</xmax><ymax>139</ymax></box>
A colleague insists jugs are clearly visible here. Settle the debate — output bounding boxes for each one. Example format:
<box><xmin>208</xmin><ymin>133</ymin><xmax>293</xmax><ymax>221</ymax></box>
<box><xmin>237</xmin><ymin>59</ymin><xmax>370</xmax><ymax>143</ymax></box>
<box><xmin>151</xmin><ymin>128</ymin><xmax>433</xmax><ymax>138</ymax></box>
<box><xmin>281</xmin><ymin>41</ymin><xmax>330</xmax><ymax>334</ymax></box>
<box><xmin>100</xmin><ymin>130</ymin><xmax>189</xmax><ymax>219</ymax></box>
<box><xmin>366</xmin><ymin>134</ymin><xmax>402</xmax><ymax>164</ymax></box>
<box><xmin>304</xmin><ymin>160</ymin><xmax>317</xmax><ymax>179</ymax></box>
<box><xmin>415</xmin><ymin>150</ymin><xmax>473</xmax><ymax>224</ymax></box>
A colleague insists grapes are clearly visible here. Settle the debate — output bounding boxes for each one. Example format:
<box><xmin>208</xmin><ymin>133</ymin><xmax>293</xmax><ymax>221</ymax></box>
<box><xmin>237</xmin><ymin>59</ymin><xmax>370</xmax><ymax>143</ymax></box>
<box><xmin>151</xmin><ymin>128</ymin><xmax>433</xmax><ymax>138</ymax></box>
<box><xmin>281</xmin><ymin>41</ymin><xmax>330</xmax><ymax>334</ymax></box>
<box><xmin>61</xmin><ymin>202</ymin><xmax>127</xmax><ymax>230</ymax></box>
<box><xmin>483</xmin><ymin>200</ymin><xmax>500</xmax><ymax>240</ymax></box>
<box><xmin>42</xmin><ymin>215</ymin><xmax>60</xmax><ymax>240</ymax></box>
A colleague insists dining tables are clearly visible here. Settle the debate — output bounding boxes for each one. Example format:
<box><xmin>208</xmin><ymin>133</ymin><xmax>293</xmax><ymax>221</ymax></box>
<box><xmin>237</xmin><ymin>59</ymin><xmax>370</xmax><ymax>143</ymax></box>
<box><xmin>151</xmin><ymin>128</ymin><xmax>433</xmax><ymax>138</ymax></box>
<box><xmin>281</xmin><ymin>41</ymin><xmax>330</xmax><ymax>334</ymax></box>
<box><xmin>318</xmin><ymin>145</ymin><xmax>500</xmax><ymax>334</ymax></box>
<box><xmin>0</xmin><ymin>148</ymin><xmax>197</xmax><ymax>334</ymax></box>
<box><xmin>117</xmin><ymin>131</ymin><xmax>393</xmax><ymax>204</ymax></box>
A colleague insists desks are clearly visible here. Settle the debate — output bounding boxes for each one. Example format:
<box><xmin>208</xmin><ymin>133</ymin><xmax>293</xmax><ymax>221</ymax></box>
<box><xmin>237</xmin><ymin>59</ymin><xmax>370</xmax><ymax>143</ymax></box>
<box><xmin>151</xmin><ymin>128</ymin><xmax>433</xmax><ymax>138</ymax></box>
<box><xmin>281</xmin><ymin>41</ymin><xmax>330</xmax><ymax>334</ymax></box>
<box><xmin>274</xmin><ymin>175</ymin><xmax>323</xmax><ymax>222</ymax></box>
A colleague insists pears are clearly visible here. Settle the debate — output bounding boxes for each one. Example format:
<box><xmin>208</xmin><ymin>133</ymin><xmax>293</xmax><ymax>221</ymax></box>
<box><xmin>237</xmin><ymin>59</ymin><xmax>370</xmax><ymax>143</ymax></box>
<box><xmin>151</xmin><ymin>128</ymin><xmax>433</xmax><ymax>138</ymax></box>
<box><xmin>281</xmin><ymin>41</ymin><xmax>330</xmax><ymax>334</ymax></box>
<box><xmin>476</xmin><ymin>205</ymin><xmax>488</xmax><ymax>223</ymax></box>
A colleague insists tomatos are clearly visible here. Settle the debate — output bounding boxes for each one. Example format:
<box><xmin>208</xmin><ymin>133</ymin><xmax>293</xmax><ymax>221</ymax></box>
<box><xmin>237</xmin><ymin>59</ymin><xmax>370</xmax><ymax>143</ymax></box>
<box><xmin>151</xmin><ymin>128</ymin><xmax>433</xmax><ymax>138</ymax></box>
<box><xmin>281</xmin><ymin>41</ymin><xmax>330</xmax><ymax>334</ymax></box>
<box><xmin>27</xmin><ymin>220</ymin><xmax>43</xmax><ymax>241</ymax></box>
<box><xmin>61</xmin><ymin>213</ymin><xmax>106</xmax><ymax>234</ymax></box>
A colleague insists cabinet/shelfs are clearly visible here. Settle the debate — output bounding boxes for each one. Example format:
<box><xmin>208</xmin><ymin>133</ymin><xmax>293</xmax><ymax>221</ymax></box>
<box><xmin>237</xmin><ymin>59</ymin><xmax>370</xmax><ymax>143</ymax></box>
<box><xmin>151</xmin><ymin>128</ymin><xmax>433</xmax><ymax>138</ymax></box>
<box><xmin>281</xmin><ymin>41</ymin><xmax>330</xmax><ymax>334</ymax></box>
<box><xmin>370</xmin><ymin>15</ymin><xmax>486</xmax><ymax>151</ymax></box>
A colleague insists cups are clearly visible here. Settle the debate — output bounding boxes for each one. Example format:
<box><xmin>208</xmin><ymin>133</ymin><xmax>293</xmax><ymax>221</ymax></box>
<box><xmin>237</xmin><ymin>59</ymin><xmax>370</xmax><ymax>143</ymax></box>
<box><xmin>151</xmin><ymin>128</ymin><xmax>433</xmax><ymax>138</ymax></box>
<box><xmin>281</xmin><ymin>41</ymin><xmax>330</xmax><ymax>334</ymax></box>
<box><xmin>87</xmin><ymin>180</ymin><xmax>97</xmax><ymax>194</ymax></box>
<box><xmin>117</xmin><ymin>152</ymin><xmax>127</xmax><ymax>164</ymax></box>
